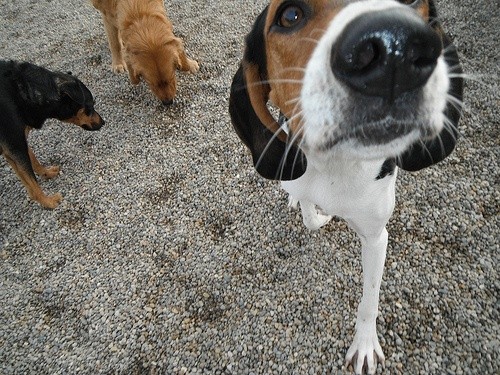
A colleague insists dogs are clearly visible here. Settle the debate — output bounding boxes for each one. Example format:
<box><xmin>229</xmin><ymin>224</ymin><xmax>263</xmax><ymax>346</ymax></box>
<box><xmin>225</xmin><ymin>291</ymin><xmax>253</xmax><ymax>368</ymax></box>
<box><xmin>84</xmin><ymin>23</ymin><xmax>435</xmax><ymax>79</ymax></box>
<box><xmin>0</xmin><ymin>59</ymin><xmax>105</xmax><ymax>210</ymax></box>
<box><xmin>228</xmin><ymin>0</ymin><xmax>492</xmax><ymax>374</ymax></box>
<box><xmin>90</xmin><ymin>0</ymin><xmax>200</xmax><ymax>107</ymax></box>
